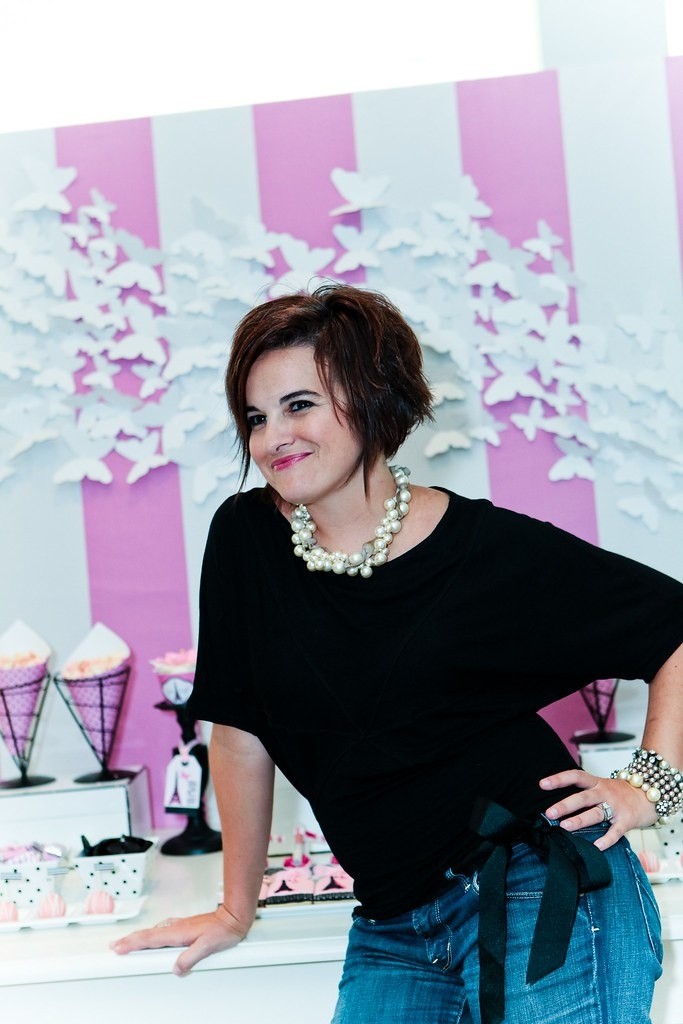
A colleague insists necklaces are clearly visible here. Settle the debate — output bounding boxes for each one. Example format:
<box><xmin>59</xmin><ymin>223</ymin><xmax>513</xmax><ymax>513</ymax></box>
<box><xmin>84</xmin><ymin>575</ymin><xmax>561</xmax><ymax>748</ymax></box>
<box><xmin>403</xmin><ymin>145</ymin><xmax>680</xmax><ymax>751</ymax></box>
<box><xmin>292</xmin><ymin>468</ymin><xmax>412</xmax><ymax>576</ymax></box>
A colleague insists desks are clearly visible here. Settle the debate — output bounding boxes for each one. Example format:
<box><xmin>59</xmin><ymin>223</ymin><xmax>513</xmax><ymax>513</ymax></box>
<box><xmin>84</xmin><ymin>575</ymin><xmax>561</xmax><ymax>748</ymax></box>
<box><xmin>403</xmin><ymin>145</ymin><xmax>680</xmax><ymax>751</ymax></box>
<box><xmin>0</xmin><ymin>830</ymin><xmax>683</xmax><ymax>1024</ymax></box>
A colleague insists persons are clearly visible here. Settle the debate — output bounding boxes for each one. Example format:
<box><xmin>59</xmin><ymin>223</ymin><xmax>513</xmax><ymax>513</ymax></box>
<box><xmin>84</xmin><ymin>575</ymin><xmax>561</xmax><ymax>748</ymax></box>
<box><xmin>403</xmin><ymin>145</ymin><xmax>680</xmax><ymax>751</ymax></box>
<box><xmin>114</xmin><ymin>283</ymin><xmax>683</xmax><ymax>1022</ymax></box>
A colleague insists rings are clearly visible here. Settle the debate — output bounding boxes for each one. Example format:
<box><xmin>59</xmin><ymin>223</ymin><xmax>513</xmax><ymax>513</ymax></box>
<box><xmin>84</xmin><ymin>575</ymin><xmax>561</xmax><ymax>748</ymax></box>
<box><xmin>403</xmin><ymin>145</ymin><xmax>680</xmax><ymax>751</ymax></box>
<box><xmin>599</xmin><ymin>802</ymin><xmax>612</xmax><ymax>821</ymax></box>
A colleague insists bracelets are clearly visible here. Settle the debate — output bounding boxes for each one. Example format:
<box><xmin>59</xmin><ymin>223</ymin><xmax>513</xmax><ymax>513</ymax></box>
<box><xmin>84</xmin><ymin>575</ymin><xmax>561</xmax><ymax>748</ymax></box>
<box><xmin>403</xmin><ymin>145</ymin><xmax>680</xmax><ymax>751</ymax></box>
<box><xmin>614</xmin><ymin>748</ymin><xmax>683</xmax><ymax>819</ymax></box>
